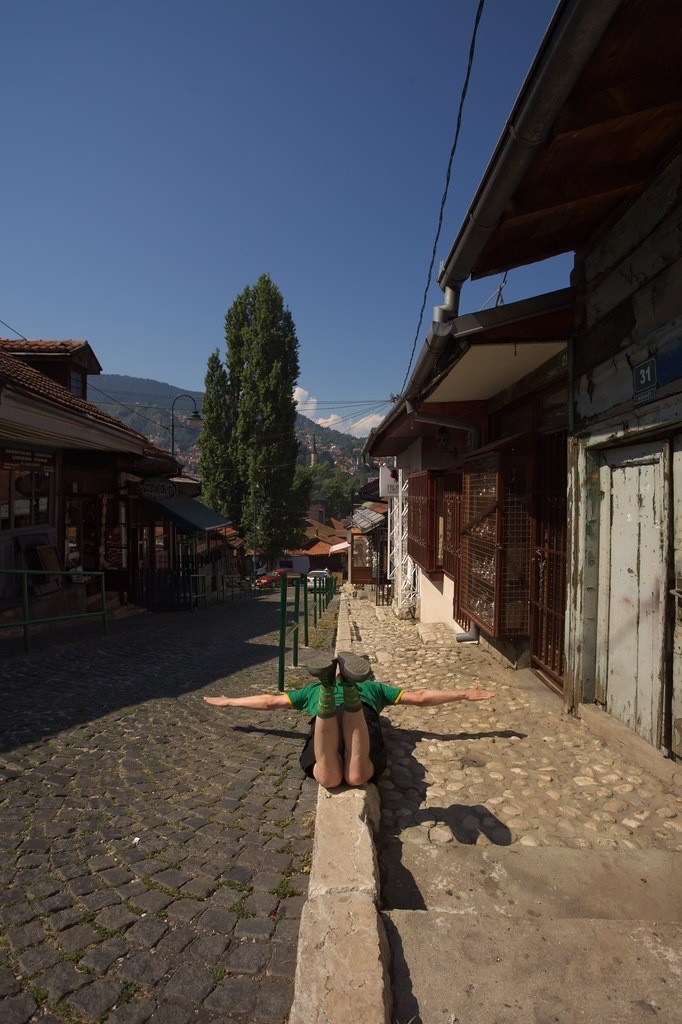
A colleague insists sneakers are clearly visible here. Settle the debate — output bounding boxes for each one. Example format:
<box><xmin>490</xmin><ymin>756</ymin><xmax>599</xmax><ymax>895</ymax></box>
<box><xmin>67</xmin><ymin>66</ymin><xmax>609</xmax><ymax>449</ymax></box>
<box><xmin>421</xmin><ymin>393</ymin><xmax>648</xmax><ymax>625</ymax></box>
<box><xmin>306</xmin><ymin>655</ymin><xmax>338</xmax><ymax>688</ymax></box>
<box><xmin>338</xmin><ymin>651</ymin><xmax>372</xmax><ymax>688</ymax></box>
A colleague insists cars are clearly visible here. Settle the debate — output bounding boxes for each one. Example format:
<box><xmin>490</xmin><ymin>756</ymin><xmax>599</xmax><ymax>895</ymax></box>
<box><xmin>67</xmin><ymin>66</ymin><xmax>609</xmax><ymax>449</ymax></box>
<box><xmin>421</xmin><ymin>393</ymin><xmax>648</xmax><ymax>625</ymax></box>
<box><xmin>306</xmin><ymin>570</ymin><xmax>332</xmax><ymax>592</ymax></box>
<box><xmin>255</xmin><ymin>569</ymin><xmax>301</xmax><ymax>588</ymax></box>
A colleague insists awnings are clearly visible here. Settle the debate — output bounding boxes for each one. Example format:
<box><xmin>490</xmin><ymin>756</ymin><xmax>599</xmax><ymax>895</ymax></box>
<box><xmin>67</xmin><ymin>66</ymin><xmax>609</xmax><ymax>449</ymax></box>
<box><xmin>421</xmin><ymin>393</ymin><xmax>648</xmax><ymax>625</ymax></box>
<box><xmin>158</xmin><ymin>496</ymin><xmax>233</xmax><ymax>533</ymax></box>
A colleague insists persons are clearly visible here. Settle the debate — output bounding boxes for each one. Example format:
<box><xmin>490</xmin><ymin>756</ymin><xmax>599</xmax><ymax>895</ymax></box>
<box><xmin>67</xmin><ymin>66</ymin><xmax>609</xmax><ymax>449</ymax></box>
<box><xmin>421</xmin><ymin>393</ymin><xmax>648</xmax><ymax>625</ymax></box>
<box><xmin>201</xmin><ymin>651</ymin><xmax>497</xmax><ymax>788</ymax></box>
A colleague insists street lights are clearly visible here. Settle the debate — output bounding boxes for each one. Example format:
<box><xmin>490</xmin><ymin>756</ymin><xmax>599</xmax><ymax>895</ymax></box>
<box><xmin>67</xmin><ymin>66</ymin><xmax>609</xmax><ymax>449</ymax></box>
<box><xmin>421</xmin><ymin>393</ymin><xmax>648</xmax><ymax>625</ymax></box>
<box><xmin>170</xmin><ymin>393</ymin><xmax>204</xmax><ymax>481</ymax></box>
<box><xmin>252</xmin><ymin>482</ymin><xmax>261</xmax><ymax>579</ymax></box>
